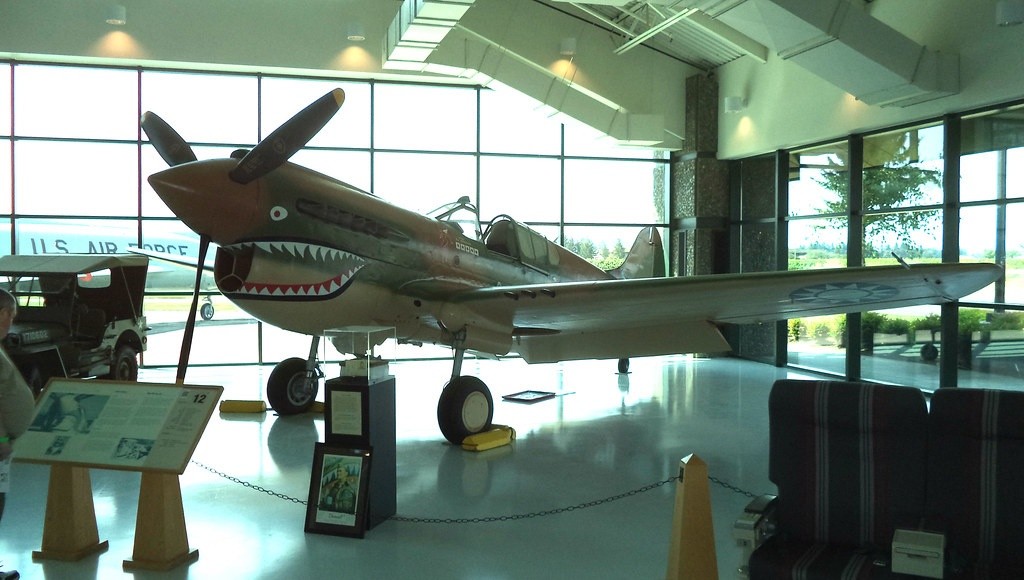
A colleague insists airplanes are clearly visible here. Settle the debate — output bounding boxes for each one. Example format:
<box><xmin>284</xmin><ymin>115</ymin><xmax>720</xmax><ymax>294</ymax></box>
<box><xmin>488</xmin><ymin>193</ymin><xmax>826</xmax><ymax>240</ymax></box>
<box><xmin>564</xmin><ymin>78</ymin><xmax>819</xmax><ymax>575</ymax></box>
<box><xmin>115</xmin><ymin>86</ymin><xmax>1002</xmax><ymax>444</ymax></box>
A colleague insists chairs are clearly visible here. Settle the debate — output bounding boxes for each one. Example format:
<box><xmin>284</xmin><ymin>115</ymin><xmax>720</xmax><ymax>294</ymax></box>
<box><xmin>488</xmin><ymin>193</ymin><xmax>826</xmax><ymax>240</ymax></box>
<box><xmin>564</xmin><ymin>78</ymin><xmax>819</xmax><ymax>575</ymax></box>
<box><xmin>731</xmin><ymin>378</ymin><xmax>929</xmax><ymax>580</ymax></box>
<box><xmin>75</xmin><ymin>309</ymin><xmax>106</xmax><ymax>349</ymax></box>
<box><xmin>924</xmin><ymin>387</ymin><xmax>1024</xmax><ymax>580</ymax></box>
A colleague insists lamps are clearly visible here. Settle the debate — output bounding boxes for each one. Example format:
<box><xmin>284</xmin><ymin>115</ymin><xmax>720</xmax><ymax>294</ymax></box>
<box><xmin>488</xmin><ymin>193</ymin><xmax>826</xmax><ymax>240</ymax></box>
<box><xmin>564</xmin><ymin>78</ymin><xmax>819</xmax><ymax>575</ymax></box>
<box><xmin>560</xmin><ymin>37</ymin><xmax>579</xmax><ymax>56</ymax></box>
<box><xmin>104</xmin><ymin>4</ymin><xmax>127</xmax><ymax>27</ymax></box>
<box><xmin>725</xmin><ymin>97</ymin><xmax>744</xmax><ymax>113</ymax></box>
<box><xmin>346</xmin><ymin>22</ymin><xmax>367</xmax><ymax>41</ymax></box>
<box><xmin>996</xmin><ymin>0</ymin><xmax>1021</xmax><ymax>27</ymax></box>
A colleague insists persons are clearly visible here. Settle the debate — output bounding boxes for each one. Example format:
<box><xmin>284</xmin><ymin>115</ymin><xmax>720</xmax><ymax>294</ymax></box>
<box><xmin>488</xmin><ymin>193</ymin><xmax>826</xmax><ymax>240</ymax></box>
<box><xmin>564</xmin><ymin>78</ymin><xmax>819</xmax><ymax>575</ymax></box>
<box><xmin>0</xmin><ymin>289</ymin><xmax>36</xmax><ymax>580</ymax></box>
<box><xmin>324</xmin><ymin>465</ymin><xmax>355</xmax><ymax>514</ymax></box>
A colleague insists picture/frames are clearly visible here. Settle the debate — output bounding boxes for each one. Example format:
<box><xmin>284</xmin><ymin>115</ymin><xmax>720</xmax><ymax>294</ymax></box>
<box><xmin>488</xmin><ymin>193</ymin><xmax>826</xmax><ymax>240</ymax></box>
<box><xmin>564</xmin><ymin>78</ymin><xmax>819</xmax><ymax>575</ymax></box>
<box><xmin>303</xmin><ymin>441</ymin><xmax>374</xmax><ymax>540</ymax></box>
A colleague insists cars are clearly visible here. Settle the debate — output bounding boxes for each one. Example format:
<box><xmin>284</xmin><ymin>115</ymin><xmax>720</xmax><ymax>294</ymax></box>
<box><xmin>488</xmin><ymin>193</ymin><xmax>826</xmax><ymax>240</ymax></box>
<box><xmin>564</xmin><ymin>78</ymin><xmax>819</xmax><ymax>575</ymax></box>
<box><xmin>0</xmin><ymin>246</ymin><xmax>149</xmax><ymax>397</ymax></box>
